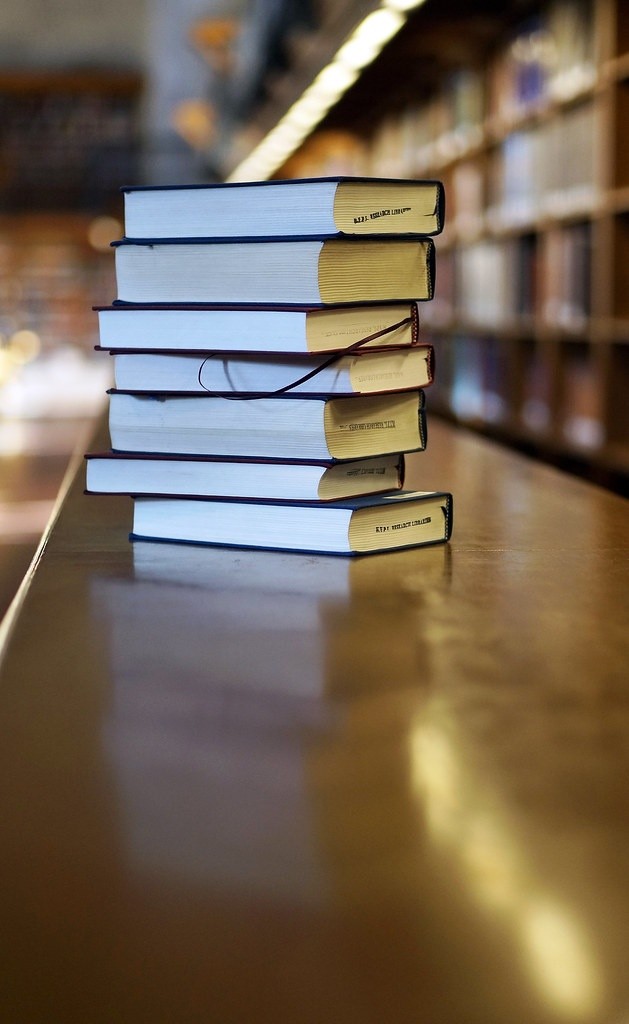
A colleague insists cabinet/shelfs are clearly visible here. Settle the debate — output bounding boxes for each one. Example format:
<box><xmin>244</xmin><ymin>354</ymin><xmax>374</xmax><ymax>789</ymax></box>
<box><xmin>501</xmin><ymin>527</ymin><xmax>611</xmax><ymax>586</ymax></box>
<box><xmin>0</xmin><ymin>1</ymin><xmax>629</xmax><ymax>482</ymax></box>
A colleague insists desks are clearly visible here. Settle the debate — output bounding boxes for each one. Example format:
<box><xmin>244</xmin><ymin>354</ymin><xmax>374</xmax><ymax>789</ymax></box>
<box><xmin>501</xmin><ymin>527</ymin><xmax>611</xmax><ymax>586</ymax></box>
<box><xmin>0</xmin><ymin>376</ymin><xmax>629</xmax><ymax>1024</ymax></box>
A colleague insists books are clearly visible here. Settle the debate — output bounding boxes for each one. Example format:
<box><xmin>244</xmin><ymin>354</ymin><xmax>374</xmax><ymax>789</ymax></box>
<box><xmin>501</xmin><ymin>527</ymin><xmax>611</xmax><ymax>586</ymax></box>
<box><xmin>109</xmin><ymin>235</ymin><xmax>436</xmax><ymax>307</ymax></box>
<box><xmin>80</xmin><ymin>300</ymin><xmax>453</xmax><ymax>558</ymax></box>
<box><xmin>119</xmin><ymin>176</ymin><xmax>446</xmax><ymax>236</ymax></box>
<box><xmin>372</xmin><ymin>10</ymin><xmax>600</xmax><ymax>455</ymax></box>
